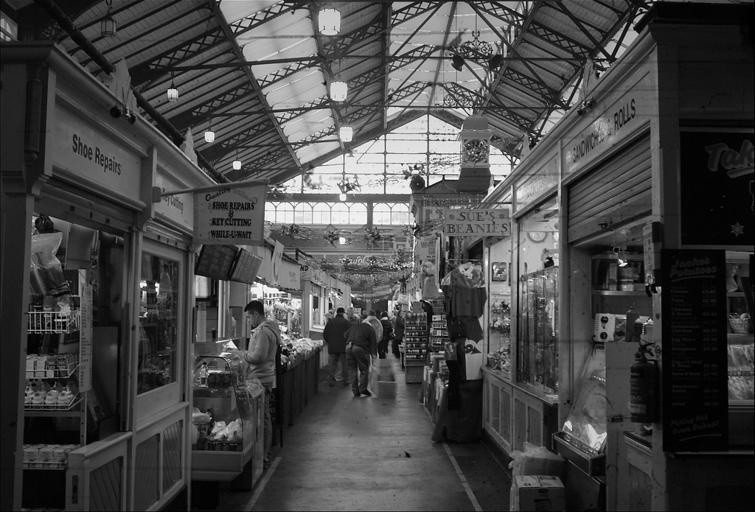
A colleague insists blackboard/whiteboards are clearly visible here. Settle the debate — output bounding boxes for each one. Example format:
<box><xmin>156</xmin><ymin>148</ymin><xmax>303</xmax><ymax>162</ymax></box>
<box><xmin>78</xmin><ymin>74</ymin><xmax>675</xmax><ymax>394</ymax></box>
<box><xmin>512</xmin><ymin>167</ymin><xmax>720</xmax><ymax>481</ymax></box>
<box><xmin>661</xmin><ymin>248</ymin><xmax>729</xmax><ymax>453</ymax></box>
<box><xmin>676</xmin><ymin>126</ymin><xmax>755</xmax><ymax>252</ymax></box>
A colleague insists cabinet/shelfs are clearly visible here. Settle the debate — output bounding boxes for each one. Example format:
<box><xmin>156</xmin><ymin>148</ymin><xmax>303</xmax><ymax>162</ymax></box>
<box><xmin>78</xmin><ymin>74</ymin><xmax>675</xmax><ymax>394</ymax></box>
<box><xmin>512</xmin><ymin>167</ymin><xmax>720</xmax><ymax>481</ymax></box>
<box><xmin>190</xmin><ymin>349</ymin><xmax>258</xmax><ymax>481</ymax></box>
<box><xmin>21</xmin><ymin>295</ymin><xmax>89</xmax><ymax>471</ymax></box>
<box><xmin>404</xmin><ymin>313</ymin><xmax>428</xmax><ymax>385</ymax></box>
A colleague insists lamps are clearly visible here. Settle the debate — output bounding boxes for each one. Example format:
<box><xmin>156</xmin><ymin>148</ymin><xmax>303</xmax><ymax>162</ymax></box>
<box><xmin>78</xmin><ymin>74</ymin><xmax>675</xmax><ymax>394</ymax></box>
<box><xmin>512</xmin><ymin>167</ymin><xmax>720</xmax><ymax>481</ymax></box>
<box><xmin>315</xmin><ymin>4</ymin><xmax>349</xmax><ymax>102</ymax></box>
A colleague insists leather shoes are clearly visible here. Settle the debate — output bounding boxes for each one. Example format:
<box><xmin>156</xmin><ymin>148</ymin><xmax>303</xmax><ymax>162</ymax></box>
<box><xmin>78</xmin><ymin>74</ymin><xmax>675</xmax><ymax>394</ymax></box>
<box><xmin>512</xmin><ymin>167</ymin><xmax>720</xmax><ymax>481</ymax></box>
<box><xmin>353</xmin><ymin>389</ymin><xmax>371</xmax><ymax>397</ymax></box>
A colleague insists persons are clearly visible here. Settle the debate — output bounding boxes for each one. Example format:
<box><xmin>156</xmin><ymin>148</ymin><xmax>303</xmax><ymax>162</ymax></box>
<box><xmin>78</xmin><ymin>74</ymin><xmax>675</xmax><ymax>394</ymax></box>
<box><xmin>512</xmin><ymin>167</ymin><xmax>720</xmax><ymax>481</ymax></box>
<box><xmin>343</xmin><ymin>321</ymin><xmax>379</xmax><ymax>400</ymax></box>
<box><xmin>346</xmin><ymin>308</ymin><xmax>360</xmax><ymax>322</ymax></box>
<box><xmin>363</xmin><ymin>308</ymin><xmax>405</xmax><ymax>372</ymax></box>
<box><xmin>224</xmin><ymin>301</ymin><xmax>282</xmax><ymax>465</ymax></box>
<box><xmin>323</xmin><ymin>307</ymin><xmax>351</xmax><ymax>388</ymax></box>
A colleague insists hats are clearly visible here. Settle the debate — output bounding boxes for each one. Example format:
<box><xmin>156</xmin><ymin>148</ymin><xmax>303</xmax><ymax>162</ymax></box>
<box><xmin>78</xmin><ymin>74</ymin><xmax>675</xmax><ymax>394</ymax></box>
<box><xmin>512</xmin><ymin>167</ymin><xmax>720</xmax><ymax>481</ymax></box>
<box><xmin>337</xmin><ymin>307</ymin><xmax>346</xmax><ymax>314</ymax></box>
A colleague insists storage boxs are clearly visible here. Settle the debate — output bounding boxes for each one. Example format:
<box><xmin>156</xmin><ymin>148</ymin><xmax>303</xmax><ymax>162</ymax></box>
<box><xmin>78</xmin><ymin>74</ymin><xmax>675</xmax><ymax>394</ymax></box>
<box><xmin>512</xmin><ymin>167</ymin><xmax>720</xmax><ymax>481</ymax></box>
<box><xmin>515</xmin><ymin>472</ymin><xmax>565</xmax><ymax>512</ymax></box>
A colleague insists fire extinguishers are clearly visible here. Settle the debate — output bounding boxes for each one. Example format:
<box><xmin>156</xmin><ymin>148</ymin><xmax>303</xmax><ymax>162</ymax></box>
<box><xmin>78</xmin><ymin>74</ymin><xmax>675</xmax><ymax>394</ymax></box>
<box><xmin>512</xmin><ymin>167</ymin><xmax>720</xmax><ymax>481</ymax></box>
<box><xmin>630</xmin><ymin>343</ymin><xmax>659</xmax><ymax>423</ymax></box>
